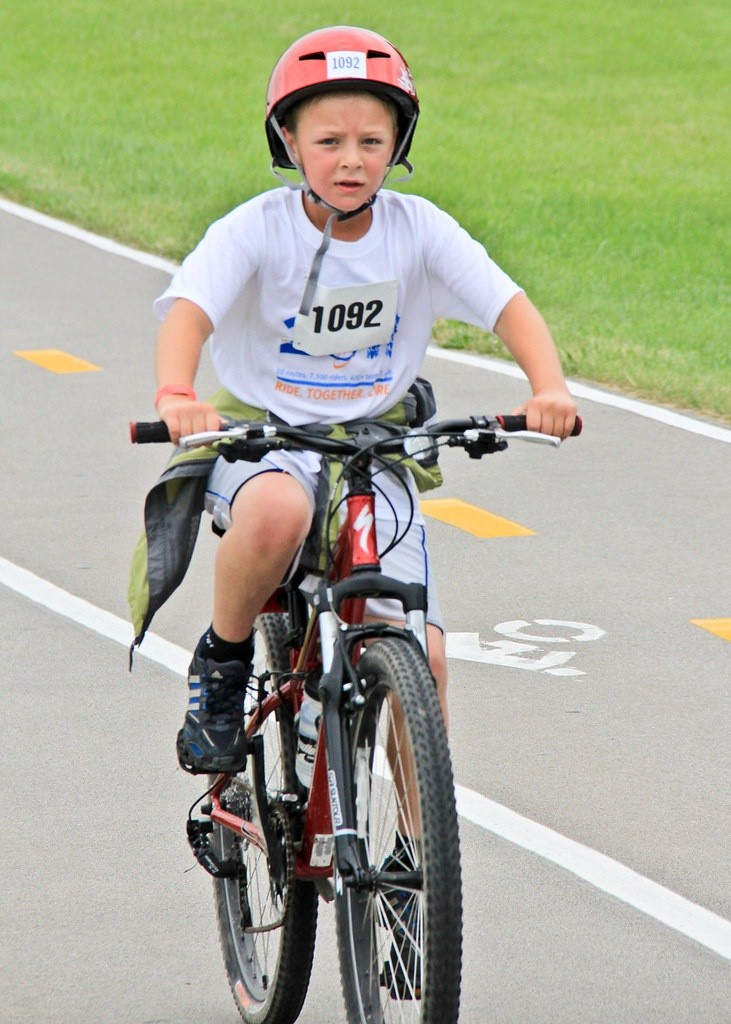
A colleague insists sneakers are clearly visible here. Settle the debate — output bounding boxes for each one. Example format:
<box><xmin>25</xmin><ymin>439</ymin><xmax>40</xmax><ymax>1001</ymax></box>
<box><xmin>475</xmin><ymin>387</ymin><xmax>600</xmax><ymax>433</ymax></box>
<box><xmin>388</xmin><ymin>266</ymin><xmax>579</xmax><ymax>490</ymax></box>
<box><xmin>378</xmin><ymin>855</ymin><xmax>425</xmax><ymax>1001</ymax></box>
<box><xmin>176</xmin><ymin>645</ymin><xmax>254</xmax><ymax>776</ymax></box>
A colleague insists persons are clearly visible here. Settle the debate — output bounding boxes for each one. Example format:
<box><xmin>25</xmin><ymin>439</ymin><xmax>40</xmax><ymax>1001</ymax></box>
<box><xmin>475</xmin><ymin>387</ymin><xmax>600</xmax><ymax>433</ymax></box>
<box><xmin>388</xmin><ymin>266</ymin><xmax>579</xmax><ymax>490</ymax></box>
<box><xmin>154</xmin><ymin>24</ymin><xmax>577</xmax><ymax>1003</ymax></box>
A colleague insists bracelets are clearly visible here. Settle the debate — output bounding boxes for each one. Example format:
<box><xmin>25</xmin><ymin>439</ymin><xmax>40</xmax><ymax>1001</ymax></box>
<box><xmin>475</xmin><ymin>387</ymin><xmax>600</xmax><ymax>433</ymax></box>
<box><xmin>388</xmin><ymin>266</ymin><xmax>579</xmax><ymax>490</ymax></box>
<box><xmin>154</xmin><ymin>383</ymin><xmax>195</xmax><ymax>406</ymax></box>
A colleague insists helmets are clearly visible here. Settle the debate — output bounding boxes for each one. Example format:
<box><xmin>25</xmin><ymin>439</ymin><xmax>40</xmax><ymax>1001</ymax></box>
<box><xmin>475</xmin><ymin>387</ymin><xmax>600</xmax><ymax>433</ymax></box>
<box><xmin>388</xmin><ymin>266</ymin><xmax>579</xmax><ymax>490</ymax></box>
<box><xmin>264</xmin><ymin>28</ymin><xmax>420</xmax><ymax>163</ymax></box>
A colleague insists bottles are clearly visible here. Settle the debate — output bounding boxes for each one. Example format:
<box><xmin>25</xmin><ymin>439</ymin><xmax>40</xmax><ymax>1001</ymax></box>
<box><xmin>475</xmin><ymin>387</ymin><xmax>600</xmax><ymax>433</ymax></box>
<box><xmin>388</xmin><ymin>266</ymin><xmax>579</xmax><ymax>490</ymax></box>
<box><xmin>295</xmin><ymin>663</ymin><xmax>326</xmax><ymax>790</ymax></box>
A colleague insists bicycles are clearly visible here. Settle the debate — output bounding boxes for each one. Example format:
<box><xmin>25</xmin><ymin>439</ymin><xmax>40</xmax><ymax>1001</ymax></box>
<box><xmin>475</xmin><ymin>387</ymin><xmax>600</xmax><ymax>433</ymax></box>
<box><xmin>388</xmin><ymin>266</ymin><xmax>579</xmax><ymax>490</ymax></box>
<box><xmin>126</xmin><ymin>411</ymin><xmax>586</xmax><ymax>1024</ymax></box>
